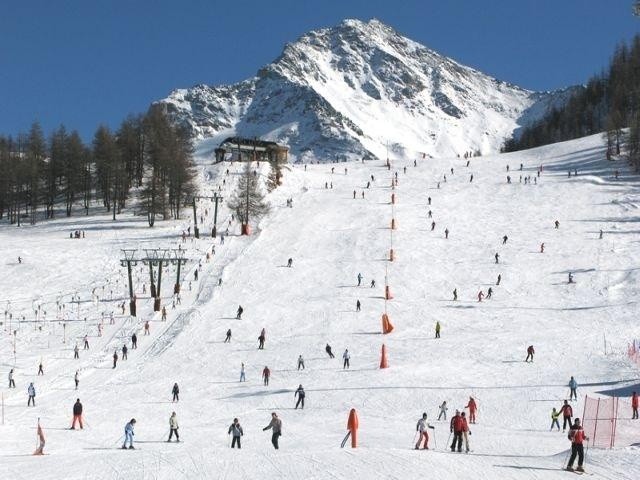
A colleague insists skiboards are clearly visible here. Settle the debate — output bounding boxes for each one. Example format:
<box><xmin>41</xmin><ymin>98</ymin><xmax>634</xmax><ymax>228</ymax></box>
<box><xmin>562</xmin><ymin>467</ymin><xmax>594</xmax><ymax>476</ymax></box>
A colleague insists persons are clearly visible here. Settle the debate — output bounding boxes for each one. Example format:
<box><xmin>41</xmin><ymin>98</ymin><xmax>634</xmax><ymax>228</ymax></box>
<box><xmin>631</xmin><ymin>390</ymin><xmax>639</xmax><ymax>421</ymax></box>
<box><xmin>286</xmin><ymin>152</ymin><xmax>620</xmax><ymax>363</ymax></box>
<box><xmin>414</xmin><ymin>395</ymin><xmax>478</xmax><ymax>453</ymax></box>
<box><xmin>8</xmin><ymin>168</ymin><xmax>235</xmax><ymax>450</ymax></box>
<box><xmin>550</xmin><ymin>376</ymin><xmax>589</xmax><ymax>472</ymax></box>
<box><xmin>223</xmin><ymin>305</ymin><xmax>305</xmax><ymax>450</ymax></box>
